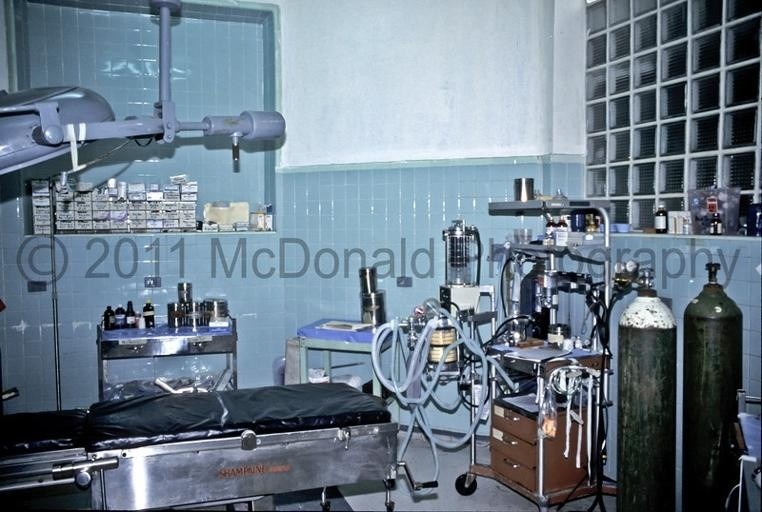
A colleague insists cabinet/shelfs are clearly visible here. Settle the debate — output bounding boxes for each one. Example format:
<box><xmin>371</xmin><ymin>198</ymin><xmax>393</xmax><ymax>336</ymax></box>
<box><xmin>97</xmin><ymin>320</ymin><xmax>398</xmax><ymax>427</ymax></box>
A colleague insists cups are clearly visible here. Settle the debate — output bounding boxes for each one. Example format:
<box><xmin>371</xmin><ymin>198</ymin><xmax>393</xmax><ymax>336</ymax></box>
<box><xmin>514</xmin><ymin>177</ymin><xmax>536</xmax><ymax>200</ymax></box>
<box><xmin>514</xmin><ymin>229</ymin><xmax>533</xmax><ymax>245</ymax></box>
<box><xmin>746</xmin><ymin>203</ymin><xmax>762</xmax><ymax>237</ymax></box>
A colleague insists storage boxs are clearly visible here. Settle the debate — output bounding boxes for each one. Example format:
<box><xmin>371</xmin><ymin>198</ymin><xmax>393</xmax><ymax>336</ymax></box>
<box><xmin>491</xmin><ymin>392</ymin><xmax>588</xmax><ymax>491</ymax></box>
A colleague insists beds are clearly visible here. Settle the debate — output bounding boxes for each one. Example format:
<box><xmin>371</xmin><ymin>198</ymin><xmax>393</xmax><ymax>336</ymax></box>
<box><xmin>0</xmin><ymin>382</ymin><xmax>397</xmax><ymax>512</ymax></box>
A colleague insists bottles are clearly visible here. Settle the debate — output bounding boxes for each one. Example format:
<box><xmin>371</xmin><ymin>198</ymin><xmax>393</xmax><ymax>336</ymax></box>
<box><xmin>708</xmin><ymin>212</ymin><xmax>724</xmax><ymax>236</ymax></box>
<box><xmin>167</xmin><ymin>282</ymin><xmax>226</xmax><ymax>330</ymax></box>
<box><xmin>653</xmin><ymin>204</ymin><xmax>669</xmax><ymax>233</ymax></box>
<box><xmin>102</xmin><ymin>299</ymin><xmax>156</xmax><ymax>331</ymax></box>
<box><xmin>358</xmin><ymin>266</ymin><xmax>386</xmax><ymax>325</ymax></box>
<box><xmin>545</xmin><ymin>216</ymin><xmax>569</xmax><ymax>241</ymax></box>
